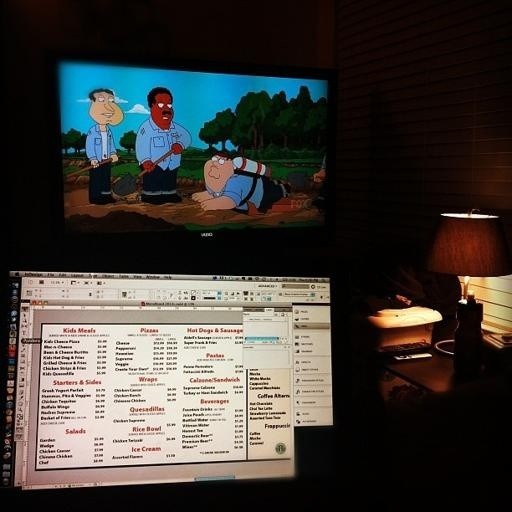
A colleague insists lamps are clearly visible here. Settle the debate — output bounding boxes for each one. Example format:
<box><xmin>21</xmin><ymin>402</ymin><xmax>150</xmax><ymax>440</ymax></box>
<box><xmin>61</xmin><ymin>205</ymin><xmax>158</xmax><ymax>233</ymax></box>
<box><xmin>423</xmin><ymin>211</ymin><xmax>512</xmax><ymax>358</ymax></box>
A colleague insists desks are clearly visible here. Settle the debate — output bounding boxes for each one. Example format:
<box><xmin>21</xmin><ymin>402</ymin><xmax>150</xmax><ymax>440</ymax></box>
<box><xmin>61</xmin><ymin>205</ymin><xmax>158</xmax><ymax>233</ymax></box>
<box><xmin>352</xmin><ymin>326</ymin><xmax>455</xmax><ymax>400</ymax></box>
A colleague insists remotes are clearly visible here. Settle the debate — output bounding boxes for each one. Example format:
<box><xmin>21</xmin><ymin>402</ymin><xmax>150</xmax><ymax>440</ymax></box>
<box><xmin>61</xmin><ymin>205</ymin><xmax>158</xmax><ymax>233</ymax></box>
<box><xmin>393</xmin><ymin>352</ymin><xmax>433</xmax><ymax>361</ymax></box>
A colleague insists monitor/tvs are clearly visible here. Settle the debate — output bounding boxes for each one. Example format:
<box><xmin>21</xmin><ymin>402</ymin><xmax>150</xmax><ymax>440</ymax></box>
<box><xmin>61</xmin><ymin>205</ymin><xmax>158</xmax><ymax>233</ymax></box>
<box><xmin>0</xmin><ymin>258</ymin><xmax>342</xmax><ymax>503</ymax></box>
<box><xmin>36</xmin><ymin>47</ymin><xmax>340</xmax><ymax>255</ymax></box>
